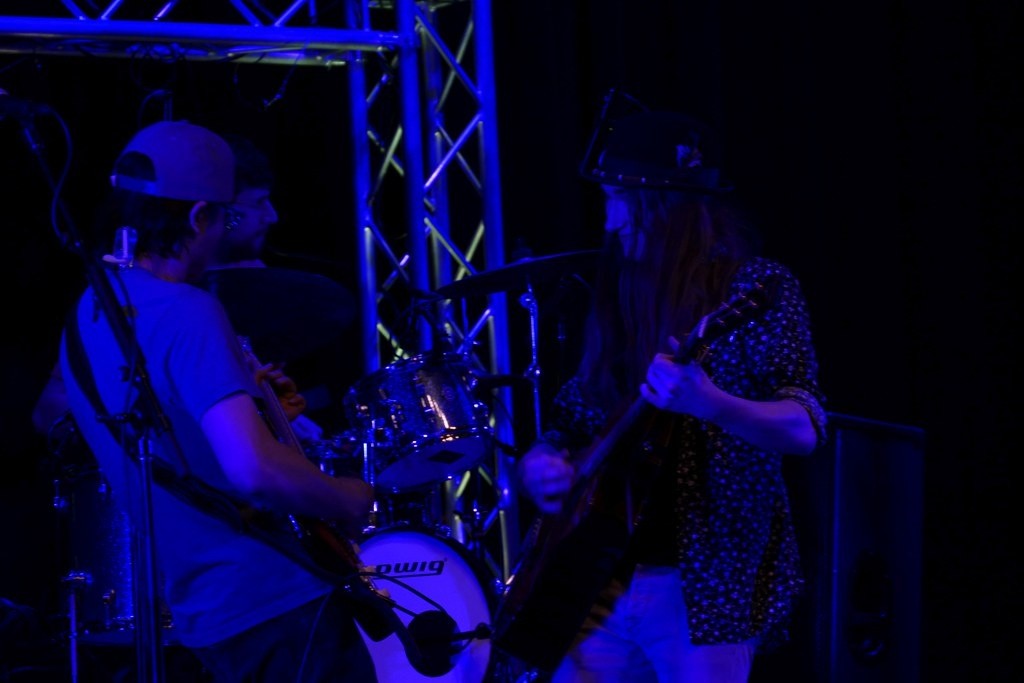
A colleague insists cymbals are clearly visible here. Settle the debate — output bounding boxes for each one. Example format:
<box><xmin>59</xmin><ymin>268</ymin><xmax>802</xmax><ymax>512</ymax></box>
<box><xmin>437</xmin><ymin>247</ymin><xmax>609</xmax><ymax>304</ymax></box>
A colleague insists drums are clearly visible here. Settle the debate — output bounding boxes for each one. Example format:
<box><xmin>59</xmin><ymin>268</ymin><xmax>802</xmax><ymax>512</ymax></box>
<box><xmin>346</xmin><ymin>527</ymin><xmax>501</xmax><ymax>683</ymax></box>
<box><xmin>338</xmin><ymin>346</ymin><xmax>500</xmax><ymax>499</ymax></box>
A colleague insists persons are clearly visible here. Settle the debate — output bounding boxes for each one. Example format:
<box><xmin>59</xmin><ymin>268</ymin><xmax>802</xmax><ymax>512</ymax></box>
<box><xmin>208</xmin><ymin>146</ymin><xmax>282</xmax><ymax>266</ymax></box>
<box><xmin>516</xmin><ymin>115</ymin><xmax>835</xmax><ymax>683</ymax></box>
<box><xmin>40</xmin><ymin>120</ymin><xmax>395</xmax><ymax>682</ymax></box>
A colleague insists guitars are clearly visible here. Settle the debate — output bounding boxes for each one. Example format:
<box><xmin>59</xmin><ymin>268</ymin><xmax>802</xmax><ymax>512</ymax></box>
<box><xmin>233</xmin><ymin>330</ymin><xmax>401</xmax><ymax>646</ymax></box>
<box><xmin>485</xmin><ymin>262</ymin><xmax>785</xmax><ymax>682</ymax></box>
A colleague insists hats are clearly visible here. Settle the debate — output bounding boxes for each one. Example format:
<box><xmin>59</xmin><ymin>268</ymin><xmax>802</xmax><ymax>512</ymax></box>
<box><xmin>582</xmin><ymin>102</ymin><xmax>724</xmax><ymax>197</ymax></box>
<box><xmin>109</xmin><ymin>118</ymin><xmax>235</xmax><ymax>208</ymax></box>
